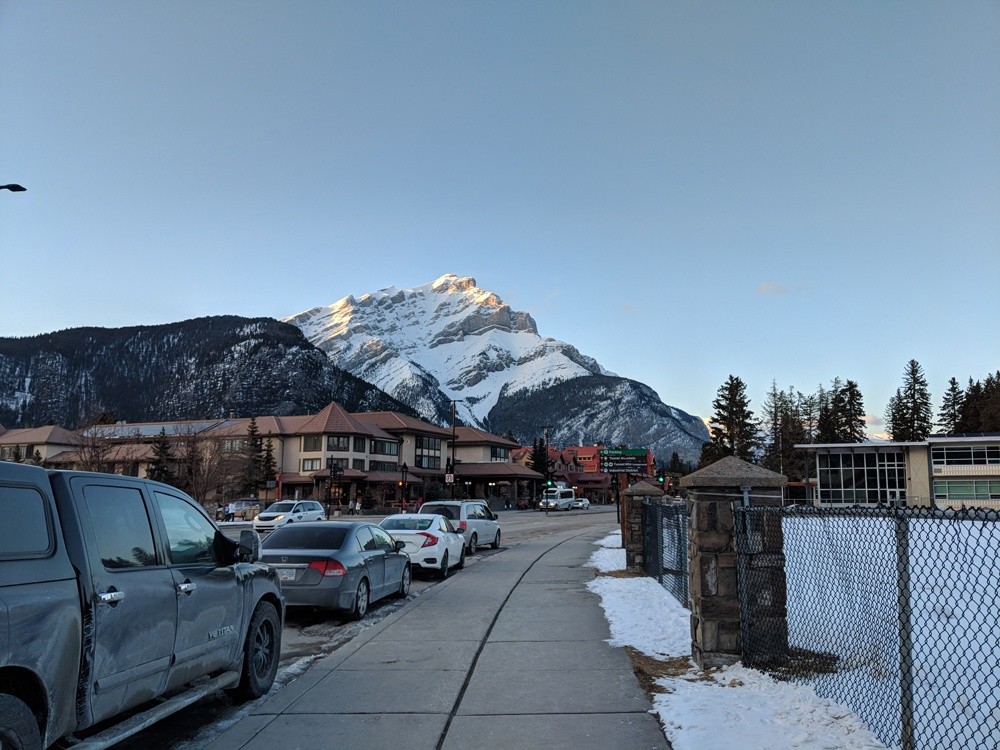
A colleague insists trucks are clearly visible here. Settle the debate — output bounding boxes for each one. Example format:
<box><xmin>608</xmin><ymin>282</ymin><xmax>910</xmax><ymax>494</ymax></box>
<box><xmin>538</xmin><ymin>488</ymin><xmax>575</xmax><ymax>511</ymax></box>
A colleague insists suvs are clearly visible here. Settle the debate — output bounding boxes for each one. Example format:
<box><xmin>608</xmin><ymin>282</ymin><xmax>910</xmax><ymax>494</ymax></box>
<box><xmin>416</xmin><ymin>499</ymin><xmax>501</xmax><ymax>555</ymax></box>
<box><xmin>215</xmin><ymin>498</ymin><xmax>277</xmax><ymax>521</ymax></box>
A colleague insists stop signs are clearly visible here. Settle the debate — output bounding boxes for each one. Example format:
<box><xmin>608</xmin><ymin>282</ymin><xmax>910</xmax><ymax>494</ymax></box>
<box><xmin>336</xmin><ymin>467</ymin><xmax>345</xmax><ymax>479</ymax></box>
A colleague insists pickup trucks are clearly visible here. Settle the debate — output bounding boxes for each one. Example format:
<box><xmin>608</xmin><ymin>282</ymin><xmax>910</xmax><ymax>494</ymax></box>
<box><xmin>0</xmin><ymin>460</ymin><xmax>288</xmax><ymax>750</ymax></box>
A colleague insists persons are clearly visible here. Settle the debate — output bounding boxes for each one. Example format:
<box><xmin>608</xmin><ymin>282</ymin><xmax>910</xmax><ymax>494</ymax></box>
<box><xmin>356</xmin><ymin>500</ymin><xmax>361</xmax><ymax>515</ymax></box>
<box><xmin>505</xmin><ymin>497</ymin><xmax>510</xmax><ymax>510</ymax></box>
<box><xmin>224</xmin><ymin>502</ymin><xmax>236</xmax><ymax>522</ymax></box>
<box><xmin>347</xmin><ymin>499</ymin><xmax>354</xmax><ymax>516</ymax></box>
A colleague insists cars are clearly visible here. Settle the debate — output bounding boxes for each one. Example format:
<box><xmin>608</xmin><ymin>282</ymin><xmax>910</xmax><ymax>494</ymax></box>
<box><xmin>258</xmin><ymin>520</ymin><xmax>413</xmax><ymax>621</ymax></box>
<box><xmin>572</xmin><ymin>498</ymin><xmax>591</xmax><ymax>510</ymax></box>
<box><xmin>253</xmin><ymin>498</ymin><xmax>327</xmax><ymax>533</ymax></box>
<box><xmin>377</xmin><ymin>514</ymin><xmax>466</xmax><ymax>580</ymax></box>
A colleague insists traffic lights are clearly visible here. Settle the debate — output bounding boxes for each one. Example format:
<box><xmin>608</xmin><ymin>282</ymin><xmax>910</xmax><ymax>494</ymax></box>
<box><xmin>547</xmin><ymin>472</ymin><xmax>553</xmax><ymax>486</ymax></box>
<box><xmin>656</xmin><ymin>469</ymin><xmax>665</xmax><ymax>483</ymax></box>
<box><xmin>398</xmin><ymin>481</ymin><xmax>404</xmax><ymax>490</ymax></box>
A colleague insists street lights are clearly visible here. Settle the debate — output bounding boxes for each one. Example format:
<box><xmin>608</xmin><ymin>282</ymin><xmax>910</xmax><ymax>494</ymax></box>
<box><xmin>446</xmin><ymin>399</ymin><xmax>464</xmax><ymax>501</ymax></box>
<box><xmin>539</xmin><ymin>425</ymin><xmax>554</xmax><ymax>516</ymax></box>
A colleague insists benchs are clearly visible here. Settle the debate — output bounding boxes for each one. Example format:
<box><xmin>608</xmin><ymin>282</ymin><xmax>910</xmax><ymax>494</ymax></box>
<box><xmin>235</xmin><ymin>510</ymin><xmax>246</xmax><ymax>520</ymax></box>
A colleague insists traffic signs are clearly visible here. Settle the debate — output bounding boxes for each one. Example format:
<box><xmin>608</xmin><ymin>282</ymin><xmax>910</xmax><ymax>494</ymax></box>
<box><xmin>599</xmin><ymin>450</ymin><xmax>647</xmax><ymax>474</ymax></box>
